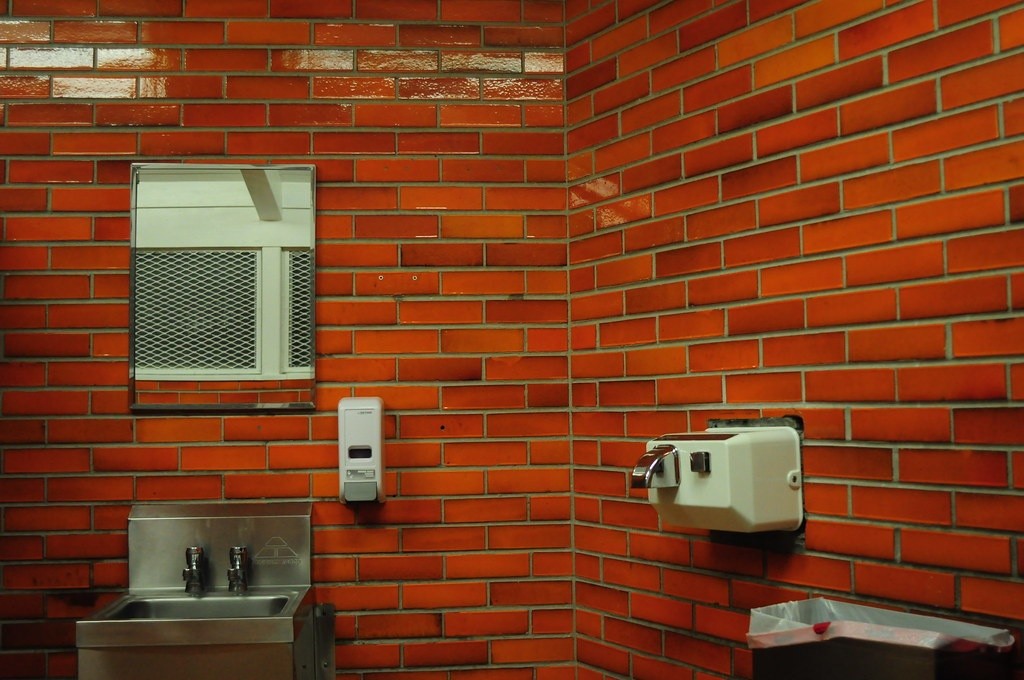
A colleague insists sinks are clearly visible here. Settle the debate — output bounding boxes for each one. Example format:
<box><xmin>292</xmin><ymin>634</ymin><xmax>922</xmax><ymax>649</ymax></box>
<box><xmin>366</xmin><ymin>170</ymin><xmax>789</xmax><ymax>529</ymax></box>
<box><xmin>99</xmin><ymin>596</ymin><xmax>289</xmax><ymax>619</ymax></box>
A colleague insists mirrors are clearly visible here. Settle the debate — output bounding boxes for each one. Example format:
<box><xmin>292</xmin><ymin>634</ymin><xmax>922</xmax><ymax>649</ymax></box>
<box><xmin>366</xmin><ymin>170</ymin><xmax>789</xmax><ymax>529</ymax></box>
<box><xmin>124</xmin><ymin>161</ymin><xmax>319</xmax><ymax>415</ymax></box>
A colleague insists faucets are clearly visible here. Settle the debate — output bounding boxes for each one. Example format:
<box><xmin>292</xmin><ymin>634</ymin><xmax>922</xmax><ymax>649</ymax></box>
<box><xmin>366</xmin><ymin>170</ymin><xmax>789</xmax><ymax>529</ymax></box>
<box><xmin>226</xmin><ymin>546</ymin><xmax>252</xmax><ymax>593</ymax></box>
<box><xmin>183</xmin><ymin>546</ymin><xmax>211</xmax><ymax>597</ymax></box>
